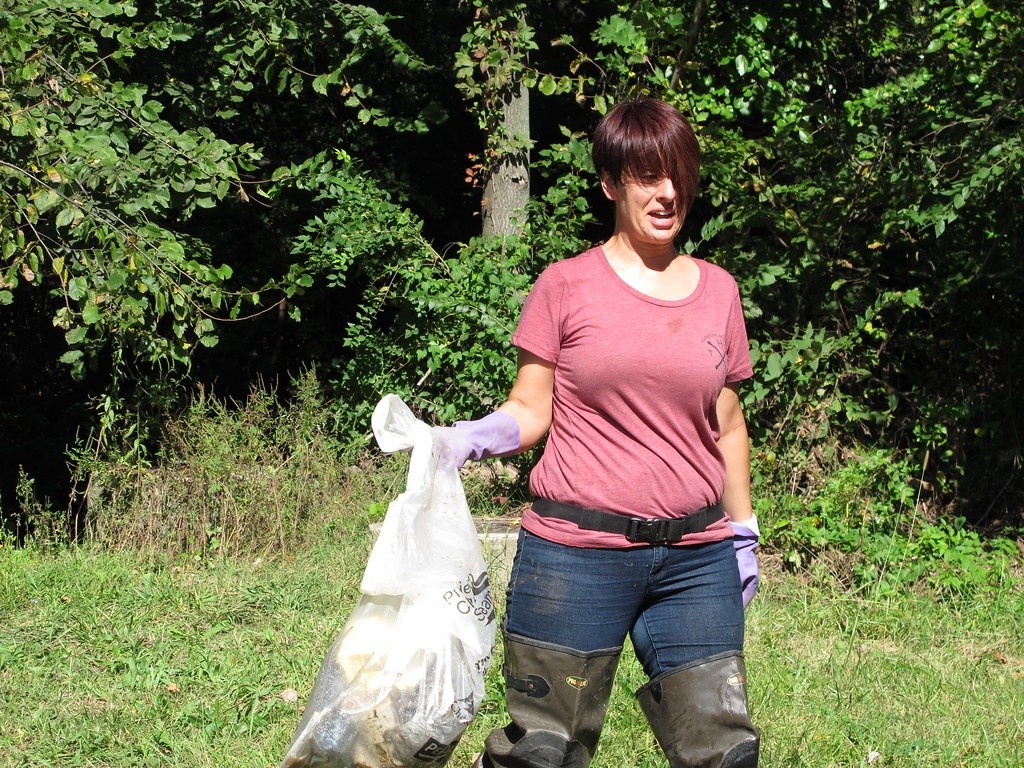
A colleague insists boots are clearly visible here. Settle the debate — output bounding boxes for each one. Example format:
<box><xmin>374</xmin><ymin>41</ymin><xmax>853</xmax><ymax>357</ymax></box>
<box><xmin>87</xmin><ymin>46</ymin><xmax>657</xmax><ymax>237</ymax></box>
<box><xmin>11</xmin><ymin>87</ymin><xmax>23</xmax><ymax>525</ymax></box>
<box><xmin>634</xmin><ymin>649</ymin><xmax>761</xmax><ymax>768</ymax></box>
<box><xmin>469</xmin><ymin>613</ymin><xmax>625</xmax><ymax>768</ymax></box>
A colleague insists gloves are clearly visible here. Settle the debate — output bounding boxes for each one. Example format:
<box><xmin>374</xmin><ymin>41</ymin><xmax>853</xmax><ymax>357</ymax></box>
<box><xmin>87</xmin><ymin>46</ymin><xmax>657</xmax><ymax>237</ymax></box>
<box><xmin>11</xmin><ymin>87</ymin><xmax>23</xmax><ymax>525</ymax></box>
<box><xmin>407</xmin><ymin>411</ymin><xmax>520</xmax><ymax>471</ymax></box>
<box><xmin>728</xmin><ymin>513</ymin><xmax>760</xmax><ymax>611</ymax></box>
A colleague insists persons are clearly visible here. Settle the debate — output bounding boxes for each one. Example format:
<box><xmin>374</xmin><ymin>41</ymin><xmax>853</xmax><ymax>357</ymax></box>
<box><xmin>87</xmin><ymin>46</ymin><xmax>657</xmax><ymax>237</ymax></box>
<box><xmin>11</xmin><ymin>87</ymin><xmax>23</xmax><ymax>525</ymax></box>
<box><xmin>427</xmin><ymin>96</ymin><xmax>762</xmax><ymax>768</ymax></box>
